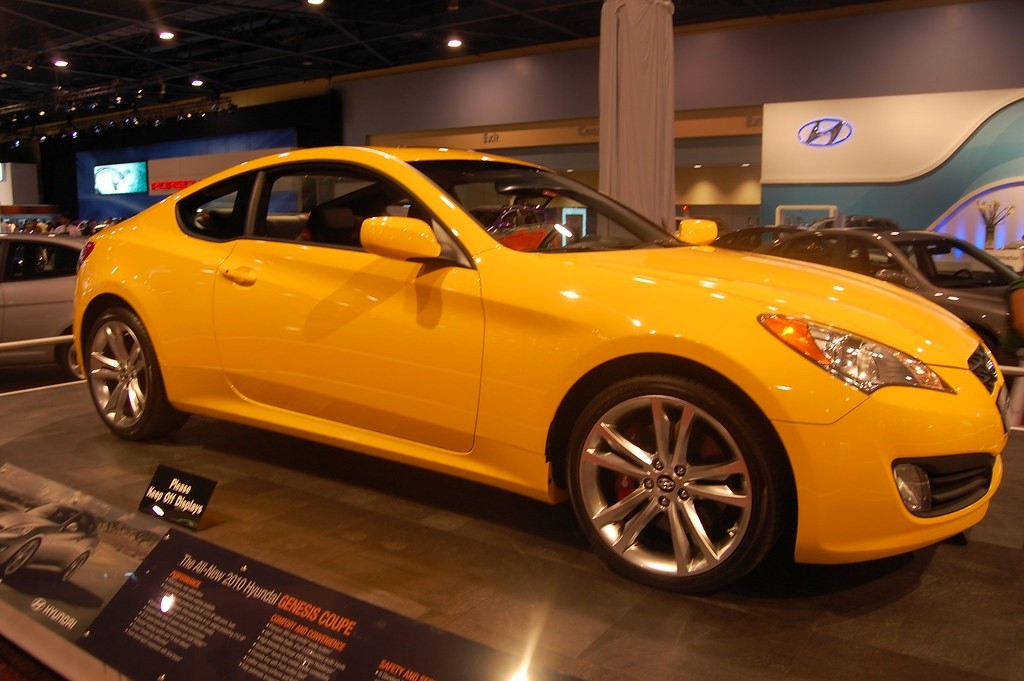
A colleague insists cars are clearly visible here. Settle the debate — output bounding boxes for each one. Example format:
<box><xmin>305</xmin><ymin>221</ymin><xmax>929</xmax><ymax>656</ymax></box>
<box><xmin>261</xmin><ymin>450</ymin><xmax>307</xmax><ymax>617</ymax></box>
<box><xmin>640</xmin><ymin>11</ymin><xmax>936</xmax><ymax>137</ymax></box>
<box><xmin>0</xmin><ymin>231</ymin><xmax>86</xmax><ymax>382</ymax></box>
<box><xmin>465</xmin><ymin>204</ymin><xmax>566</xmax><ymax>252</ymax></box>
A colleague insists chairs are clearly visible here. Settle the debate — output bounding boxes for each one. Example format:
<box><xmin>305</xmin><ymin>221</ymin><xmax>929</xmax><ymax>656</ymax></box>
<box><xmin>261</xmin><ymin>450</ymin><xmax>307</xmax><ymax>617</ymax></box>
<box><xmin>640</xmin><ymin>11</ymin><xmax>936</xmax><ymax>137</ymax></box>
<box><xmin>307</xmin><ymin>200</ymin><xmax>356</xmax><ymax>244</ymax></box>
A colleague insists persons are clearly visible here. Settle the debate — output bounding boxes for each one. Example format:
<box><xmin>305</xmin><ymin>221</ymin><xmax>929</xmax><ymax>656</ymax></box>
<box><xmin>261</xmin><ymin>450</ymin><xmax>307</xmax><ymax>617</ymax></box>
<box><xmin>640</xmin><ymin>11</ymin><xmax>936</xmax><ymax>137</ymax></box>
<box><xmin>0</xmin><ymin>212</ymin><xmax>125</xmax><ymax>237</ymax></box>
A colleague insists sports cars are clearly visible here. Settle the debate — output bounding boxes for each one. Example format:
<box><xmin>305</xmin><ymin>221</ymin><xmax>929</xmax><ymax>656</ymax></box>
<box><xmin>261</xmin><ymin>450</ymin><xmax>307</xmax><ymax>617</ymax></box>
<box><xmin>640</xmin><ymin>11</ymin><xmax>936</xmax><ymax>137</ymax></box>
<box><xmin>711</xmin><ymin>213</ymin><xmax>1023</xmax><ymax>396</ymax></box>
<box><xmin>74</xmin><ymin>143</ymin><xmax>1013</xmax><ymax>598</ymax></box>
